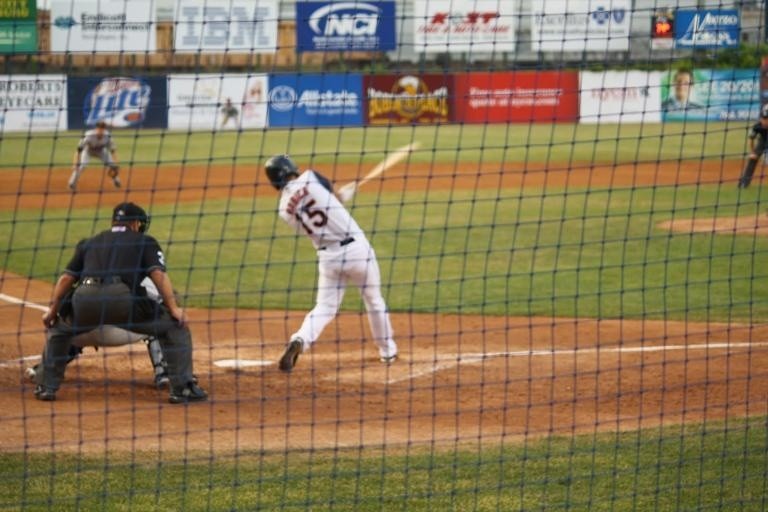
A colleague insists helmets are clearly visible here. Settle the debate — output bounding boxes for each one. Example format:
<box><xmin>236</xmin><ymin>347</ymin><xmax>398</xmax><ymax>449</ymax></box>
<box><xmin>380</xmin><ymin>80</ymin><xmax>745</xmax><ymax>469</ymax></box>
<box><xmin>114</xmin><ymin>203</ymin><xmax>150</xmax><ymax>233</ymax></box>
<box><xmin>264</xmin><ymin>154</ymin><xmax>299</xmax><ymax>190</ymax></box>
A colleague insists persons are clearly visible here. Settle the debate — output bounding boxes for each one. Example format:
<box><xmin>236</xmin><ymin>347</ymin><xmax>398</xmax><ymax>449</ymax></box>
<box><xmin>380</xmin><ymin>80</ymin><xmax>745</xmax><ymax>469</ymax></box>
<box><xmin>68</xmin><ymin>121</ymin><xmax>125</xmax><ymax>192</ymax></box>
<box><xmin>662</xmin><ymin>69</ymin><xmax>704</xmax><ymax>112</ymax></box>
<box><xmin>32</xmin><ymin>201</ymin><xmax>209</xmax><ymax>403</ymax></box>
<box><xmin>264</xmin><ymin>153</ymin><xmax>399</xmax><ymax>372</ymax></box>
<box><xmin>738</xmin><ymin>104</ymin><xmax>767</xmax><ymax>188</ymax></box>
<box><xmin>24</xmin><ymin>208</ymin><xmax>199</xmax><ymax>387</ymax></box>
<box><xmin>217</xmin><ymin>98</ymin><xmax>241</xmax><ymax>131</ymax></box>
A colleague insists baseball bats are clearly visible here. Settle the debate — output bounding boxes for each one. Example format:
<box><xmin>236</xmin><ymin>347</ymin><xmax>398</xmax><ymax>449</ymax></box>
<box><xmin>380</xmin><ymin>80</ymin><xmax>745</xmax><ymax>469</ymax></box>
<box><xmin>356</xmin><ymin>143</ymin><xmax>418</xmax><ymax>185</ymax></box>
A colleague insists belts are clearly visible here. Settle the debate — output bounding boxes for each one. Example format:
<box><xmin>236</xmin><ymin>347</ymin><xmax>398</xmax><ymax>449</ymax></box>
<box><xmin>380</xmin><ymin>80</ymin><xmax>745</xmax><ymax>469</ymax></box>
<box><xmin>320</xmin><ymin>238</ymin><xmax>355</xmax><ymax>251</ymax></box>
<box><xmin>83</xmin><ymin>275</ymin><xmax>123</xmax><ymax>285</ymax></box>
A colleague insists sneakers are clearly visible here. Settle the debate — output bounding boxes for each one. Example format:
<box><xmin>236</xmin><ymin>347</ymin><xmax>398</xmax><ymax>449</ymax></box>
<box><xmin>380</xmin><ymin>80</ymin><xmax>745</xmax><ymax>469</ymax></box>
<box><xmin>24</xmin><ymin>367</ymin><xmax>56</xmax><ymax>400</ymax></box>
<box><xmin>153</xmin><ymin>374</ymin><xmax>208</xmax><ymax>403</ymax></box>
<box><xmin>280</xmin><ymin>337</ymin><xmax>304</xmax><ymax>369</ymax></box>
